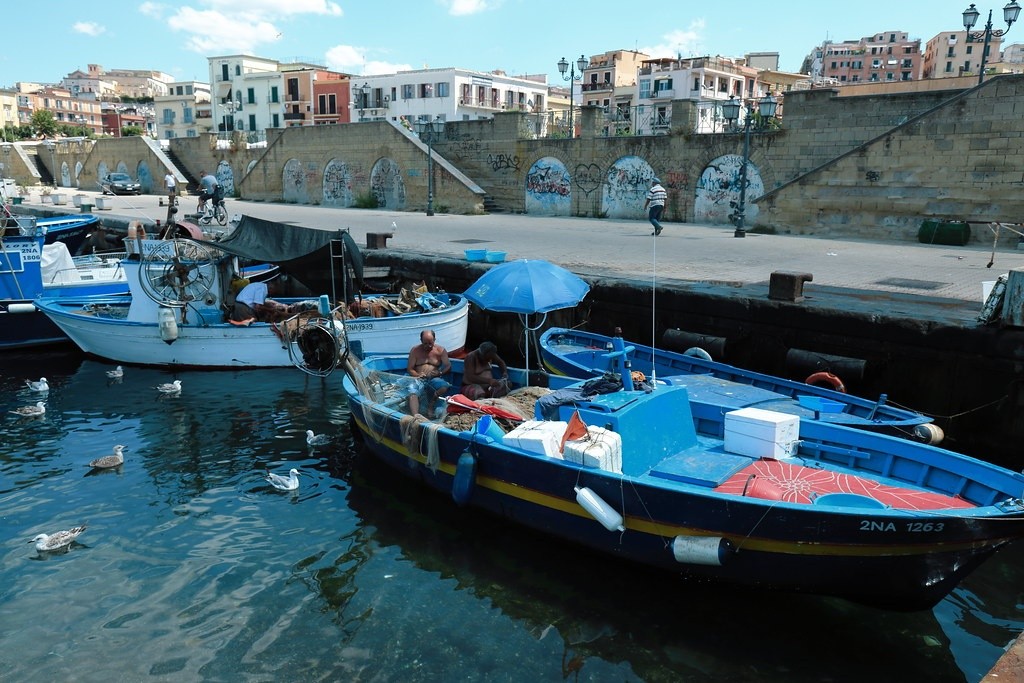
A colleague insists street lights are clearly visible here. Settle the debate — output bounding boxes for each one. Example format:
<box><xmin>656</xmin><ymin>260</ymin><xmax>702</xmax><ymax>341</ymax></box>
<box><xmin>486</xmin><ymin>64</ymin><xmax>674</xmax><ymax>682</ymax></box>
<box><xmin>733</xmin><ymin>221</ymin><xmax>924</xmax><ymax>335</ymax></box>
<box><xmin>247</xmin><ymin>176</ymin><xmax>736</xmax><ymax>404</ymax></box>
<box><xmin>722</xmin><ymin>92</ymin><xmax>778</xmax><ymax>237</ymax></box>
<box><xmin>414</xmin><ymin>114</ymin><xmax>445</xmax><ymax>216</ymax></box>
<box><xmin>141</xmin><ymin>111</ymin><xmax>150</xmax><ymax>136</ymax></box>
<box><xmin>78</xmin><ymin>119</ymin><xmax>87</xmax><ymax>139</ymax></box>
<box><xmin>226</xmin><ymin>99</ymin><xmax>240</xmax><ymax>129</ymax></box>
<box><xmin>961</xmin><ymin>0</ymin><xmax>1022</xmax><ymax>86</ymax></box>
<box><xmin>2</xmin><ymin>146</ymin><xmax>12</xmax><ymax>179</ymax></box>
<box><xmin>47</xmin><ymin>143</ymin><xmax>57</xmax><ymax>189</ymax></box>
<box><xmin>558</xmin><ymin>55</ymin><xmax>588</xmax><ymax>138</ymax></box>
<box><xmin>352</xmin><ymin>83</ymin><xmax>371</xmax><ymax>121</ymax></box>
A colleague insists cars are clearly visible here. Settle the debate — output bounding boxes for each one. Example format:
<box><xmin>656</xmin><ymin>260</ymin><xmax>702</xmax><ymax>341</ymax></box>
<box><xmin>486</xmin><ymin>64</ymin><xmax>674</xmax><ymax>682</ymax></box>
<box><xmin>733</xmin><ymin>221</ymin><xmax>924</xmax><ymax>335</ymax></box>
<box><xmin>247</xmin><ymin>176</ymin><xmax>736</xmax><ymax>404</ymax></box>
<box><xmin>103</xmin><ymin>173</ymin><xmax>141</xmax><ymax>195</ymax></box>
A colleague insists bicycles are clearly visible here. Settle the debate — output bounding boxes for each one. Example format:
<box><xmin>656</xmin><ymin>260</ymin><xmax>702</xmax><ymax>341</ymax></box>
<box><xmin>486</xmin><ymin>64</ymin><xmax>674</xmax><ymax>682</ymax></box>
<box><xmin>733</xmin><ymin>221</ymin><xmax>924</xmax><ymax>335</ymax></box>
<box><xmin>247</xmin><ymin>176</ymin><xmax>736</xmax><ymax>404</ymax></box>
<box><xmin>195</xmin><ymin>189</ymin><xmax>228</xmax><ymax>226</ymax></box>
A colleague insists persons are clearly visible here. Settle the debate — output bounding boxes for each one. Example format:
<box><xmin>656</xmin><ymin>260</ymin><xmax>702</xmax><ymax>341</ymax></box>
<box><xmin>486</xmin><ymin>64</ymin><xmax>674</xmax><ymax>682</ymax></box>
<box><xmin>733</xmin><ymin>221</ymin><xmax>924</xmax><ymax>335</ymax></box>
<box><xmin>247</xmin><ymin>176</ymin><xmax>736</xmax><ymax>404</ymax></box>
<box><xmin>233</xmin><ymin>278</ymin><xmax>278</xmax><ymax>324</ymax></box>
<box><xmin>399</xmin><ymin>115</ymin><xmax>412</xmax><ymax>131</ymax></box>
<box><xmin>644</xmin><ymin>178</ymin><xmax>668</xmax><ymax>236</ymax></box>
<box><xmin>407</xmin><ymin>330</ymin><xmax>452</xmax><ymax>420</ymax></box>
<box><xmin>195</xmin><ymin>171</ymin><xmax>220</xmax><ymax>214</ymax></box>
<box><xmin>462</xmin><ymin>342</ymin><xmax>512</xmax><ymax>400</ymax></box>
<box><xmin>163</xmin><ymin>171</ymin><xmax>176</xmax><ymax>193</ymax></box>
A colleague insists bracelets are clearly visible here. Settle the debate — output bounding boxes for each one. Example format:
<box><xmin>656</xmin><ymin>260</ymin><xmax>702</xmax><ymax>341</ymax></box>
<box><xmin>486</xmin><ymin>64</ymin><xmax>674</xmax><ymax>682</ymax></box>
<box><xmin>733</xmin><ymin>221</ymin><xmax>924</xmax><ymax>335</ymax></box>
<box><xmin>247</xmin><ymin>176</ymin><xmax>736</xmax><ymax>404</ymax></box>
<box><xmin>439</xmin><ymin>371</ymin><xmax>442</xmax><ymax>376</ymax></box>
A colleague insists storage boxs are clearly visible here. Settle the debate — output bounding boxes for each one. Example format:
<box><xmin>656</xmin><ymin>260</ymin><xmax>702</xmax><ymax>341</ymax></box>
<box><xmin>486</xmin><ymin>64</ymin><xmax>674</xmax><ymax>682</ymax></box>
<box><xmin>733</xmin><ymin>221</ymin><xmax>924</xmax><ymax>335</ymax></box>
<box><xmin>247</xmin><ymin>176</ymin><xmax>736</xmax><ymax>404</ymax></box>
<box><xmin>565</xmin><ymin>425</ymin><xmax>622</xmax><ymax>476</ymax></box>
<box><xmin>502</xmin><ymin>421</ymin><xmax>568</xmax><ymax>460</ymax></box>
<box><xmin>724</xmin><ymin>407</ymin><xmax>801</xmax><ymax>460</ymax></box>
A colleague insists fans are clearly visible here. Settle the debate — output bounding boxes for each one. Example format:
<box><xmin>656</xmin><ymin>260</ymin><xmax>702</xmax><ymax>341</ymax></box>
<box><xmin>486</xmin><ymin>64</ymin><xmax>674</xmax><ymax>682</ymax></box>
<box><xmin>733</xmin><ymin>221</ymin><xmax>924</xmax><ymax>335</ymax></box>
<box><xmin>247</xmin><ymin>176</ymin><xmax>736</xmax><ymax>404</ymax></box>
<box><xmin>144</xmin><ymin>238</ymin><xmax>215</xmax><ymax>324</ymax></box>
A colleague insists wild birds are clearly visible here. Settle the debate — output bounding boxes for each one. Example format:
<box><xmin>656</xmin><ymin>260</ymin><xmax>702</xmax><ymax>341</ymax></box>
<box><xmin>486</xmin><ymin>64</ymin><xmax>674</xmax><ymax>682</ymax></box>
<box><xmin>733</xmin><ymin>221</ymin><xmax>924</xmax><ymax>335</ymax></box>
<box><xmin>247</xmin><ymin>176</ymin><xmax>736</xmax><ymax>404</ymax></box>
<box><xmin>83</xmin><ymin>445</ymin><xmax>128</xmax><ymax>468</ymax></box>
<box><xmin>105</xmin><ymin>365</ymin><xmax>123</xmax><ymax>377</ymax></box>
<box><xmin>9</xmin><ymin>402</ymin><xmax>46</xmax><ymax>416</ymax></box>
<box><xmin>27</xmin><ymin>519</ymin><xmax>87</xmax><ymax>551</ymax></box>
<box><xmin>24</xmin><ymin>377</ymin><xmax>49</xmax><ymax>391</ymax></box>
<box><xmin>304</xmin><ymin>430</ymin><xmax>332</xmax><ymax>446</ymax></box>
<box><xmin>264</xmin><ymin>467</ymin><xmax>302</xmax><ymax>491</ymax></box>
<box><xmin>156</xmin><ymin>380</ymin><xmax>182</xmax><ymax>392</ymax></box>
<box><xmin>391</xmin><ymin>222</ymin><xmax>397</xmax><ymax>230</ymax></box>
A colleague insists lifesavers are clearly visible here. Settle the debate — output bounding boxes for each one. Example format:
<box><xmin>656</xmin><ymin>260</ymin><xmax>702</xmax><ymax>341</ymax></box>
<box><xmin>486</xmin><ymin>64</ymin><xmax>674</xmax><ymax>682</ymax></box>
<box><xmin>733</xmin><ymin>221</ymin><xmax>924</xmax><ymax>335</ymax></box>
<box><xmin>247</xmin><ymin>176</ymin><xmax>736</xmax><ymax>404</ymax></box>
<box><xmin>805</xmin><ymin>372</ymin><xmax>846</xmax><ymax>394</ymax></box>
<box><xmin>683</xmin><ymin>346</ymin><xmax>713</xmax><ymax>361</ymax></box>
<box><xmin>127</xmin><ymin>220</ymin><xmax>146</xmax><ymax>245</ymax></box>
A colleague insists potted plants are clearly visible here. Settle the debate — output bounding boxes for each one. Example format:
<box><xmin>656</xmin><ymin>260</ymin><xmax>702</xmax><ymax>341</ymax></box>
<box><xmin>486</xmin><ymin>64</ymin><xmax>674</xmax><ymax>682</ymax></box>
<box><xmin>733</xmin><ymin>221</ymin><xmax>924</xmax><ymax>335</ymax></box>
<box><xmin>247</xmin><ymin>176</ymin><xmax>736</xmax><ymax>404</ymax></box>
<box><xmin>72</xmin><ymin>194</ymin><xmax>90</xmax><ymax>208</ymax></box>
<box><xmin>16</xmin><ymin>182</ymin><xmax>34</xmax><ymax>202</ymax></box>
<box><xmin>39</xmin><ymin>185</ymin><xmax>52</xmax><ymax>203</ymax></box>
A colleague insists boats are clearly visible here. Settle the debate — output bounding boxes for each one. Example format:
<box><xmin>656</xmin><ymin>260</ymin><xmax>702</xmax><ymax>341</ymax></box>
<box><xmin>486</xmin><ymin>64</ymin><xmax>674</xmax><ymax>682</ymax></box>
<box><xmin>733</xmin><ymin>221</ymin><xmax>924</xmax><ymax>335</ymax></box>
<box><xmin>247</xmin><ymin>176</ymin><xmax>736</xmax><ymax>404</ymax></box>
<box><xmin>0</xmin><ymin>201</ymin><xmax>286</xmax><ymax>350</ymax></box>
<box><xmin>539</xmin><ymin>325</ymin><xmax>934</xmax><ymax>439</ymax></box>
<box><xmin>342</xmin><ymin>354</ymin><xmax>1024</xmax><ymax>588</ymax></box>
<box><xmin>32</xmin><ymin>189</ymin><xmax>470</xmax><ymax>370</ymax></box>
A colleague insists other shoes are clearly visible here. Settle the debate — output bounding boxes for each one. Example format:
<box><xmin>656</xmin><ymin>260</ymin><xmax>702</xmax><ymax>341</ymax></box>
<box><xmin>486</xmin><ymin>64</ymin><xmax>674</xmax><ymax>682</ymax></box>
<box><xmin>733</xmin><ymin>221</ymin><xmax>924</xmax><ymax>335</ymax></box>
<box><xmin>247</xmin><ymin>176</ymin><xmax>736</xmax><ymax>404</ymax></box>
<box><xmin>657</xmin><ymin>227</ymin><xmax>663</xmax><ymax>235</ymax></box>
<box><xmin>652</xmin><ymin>232</ymin><xmax>655</xmax><ymax>235</ymax></box>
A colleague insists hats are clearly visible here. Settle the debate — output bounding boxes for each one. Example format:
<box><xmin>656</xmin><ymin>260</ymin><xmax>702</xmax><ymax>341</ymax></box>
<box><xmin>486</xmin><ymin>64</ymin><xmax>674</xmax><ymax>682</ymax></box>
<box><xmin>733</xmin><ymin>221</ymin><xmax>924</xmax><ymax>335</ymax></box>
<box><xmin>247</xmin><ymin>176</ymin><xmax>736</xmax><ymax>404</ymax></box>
<box><xmin>652</xmin><ymin>177</ymin><xmax>661</xmax><ymax>183</ymax></box>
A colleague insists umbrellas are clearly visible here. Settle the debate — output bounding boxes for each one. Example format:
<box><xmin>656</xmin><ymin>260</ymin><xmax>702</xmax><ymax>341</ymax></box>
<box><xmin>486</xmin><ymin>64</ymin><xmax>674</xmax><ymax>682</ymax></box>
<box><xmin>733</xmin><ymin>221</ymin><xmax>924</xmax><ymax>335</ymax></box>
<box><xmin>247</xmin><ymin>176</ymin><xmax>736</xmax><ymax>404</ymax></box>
<box><xmin>462</xmin><ymin>259</ymin><xmax>592</xmax><ymax>388</ymax></box>
